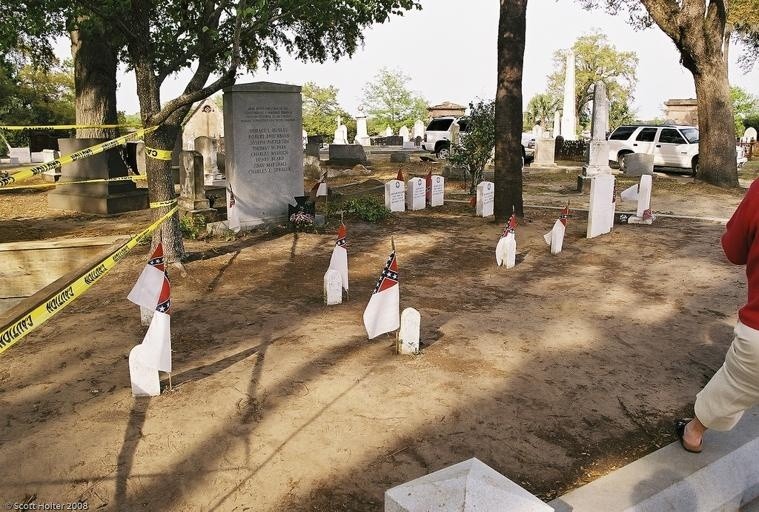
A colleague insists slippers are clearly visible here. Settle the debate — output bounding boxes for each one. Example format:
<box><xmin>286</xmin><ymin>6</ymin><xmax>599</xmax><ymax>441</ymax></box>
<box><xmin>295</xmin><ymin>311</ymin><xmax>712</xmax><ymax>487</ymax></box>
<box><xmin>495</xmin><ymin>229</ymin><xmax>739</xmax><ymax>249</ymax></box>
<box><xmin>677</xmin><ymin>417</ymin><xmax>703</xmax><ymax>453</ymax></box>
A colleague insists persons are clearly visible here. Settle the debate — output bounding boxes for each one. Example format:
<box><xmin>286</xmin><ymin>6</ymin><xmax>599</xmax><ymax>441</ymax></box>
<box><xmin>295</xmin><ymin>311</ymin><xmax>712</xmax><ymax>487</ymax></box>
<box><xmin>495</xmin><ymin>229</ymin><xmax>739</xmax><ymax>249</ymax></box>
<box><xmin>675</xmin><ymin>177</ymin><xmax>759</xmax><ymax>452</ymax></box>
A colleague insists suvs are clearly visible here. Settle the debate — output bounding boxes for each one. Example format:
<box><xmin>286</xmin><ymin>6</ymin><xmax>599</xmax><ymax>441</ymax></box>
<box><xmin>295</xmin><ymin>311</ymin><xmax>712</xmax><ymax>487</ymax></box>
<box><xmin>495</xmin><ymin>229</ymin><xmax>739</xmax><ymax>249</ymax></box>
<box><xmin>607</xmin><ymin>124</ymin><xmax>747</xmax><ymax>178</ymax></box>
<box><xmin>422</xmin><ymin>115</ymin><xmax>538</xmax><ymax>168</ymax></box>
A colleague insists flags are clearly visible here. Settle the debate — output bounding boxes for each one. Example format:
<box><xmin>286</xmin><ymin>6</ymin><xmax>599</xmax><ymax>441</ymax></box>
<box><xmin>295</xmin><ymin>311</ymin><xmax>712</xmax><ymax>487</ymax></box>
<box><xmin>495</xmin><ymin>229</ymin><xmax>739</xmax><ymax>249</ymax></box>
<box><xmin>425</xmin><ymin>171</ymin><xmax>432</xmax><ymax>202</ymax></box>
<box><xmin>134</xmin><ymin>272</ymin><xmax>172</xmax><ymax>374</ymax></box>
<box><xmin>315</xmin><ymin>173</ymin><xmax>328</xmax><ymax>197</ymax></box>
<box><xmin>125</xmin><ymin>241</ymin><xmax>164</xmax><ymax>312</ymax></box>
<box><xmin>543</xmin><ymin>204</ymin><xmax>569</xmax><ymax>245</ymax></box>
<box><xmin>328</xmin><ymin>223</ymin><xmax>349</xmax><ymax>292</ymax></box>
<box><xmin>362</xmin><ymin>249</ymin><xmax>400</xmax><ymax>339</ymax></box>
<box><xmin>397</xmin><ymin>169</ymin><xmax>404</xmax><ymax>181</ymax></box>
<box><xmin>494</xmin><ymin>213</ymin><xmax>515</xmax><ymax>266</ymax></box>
